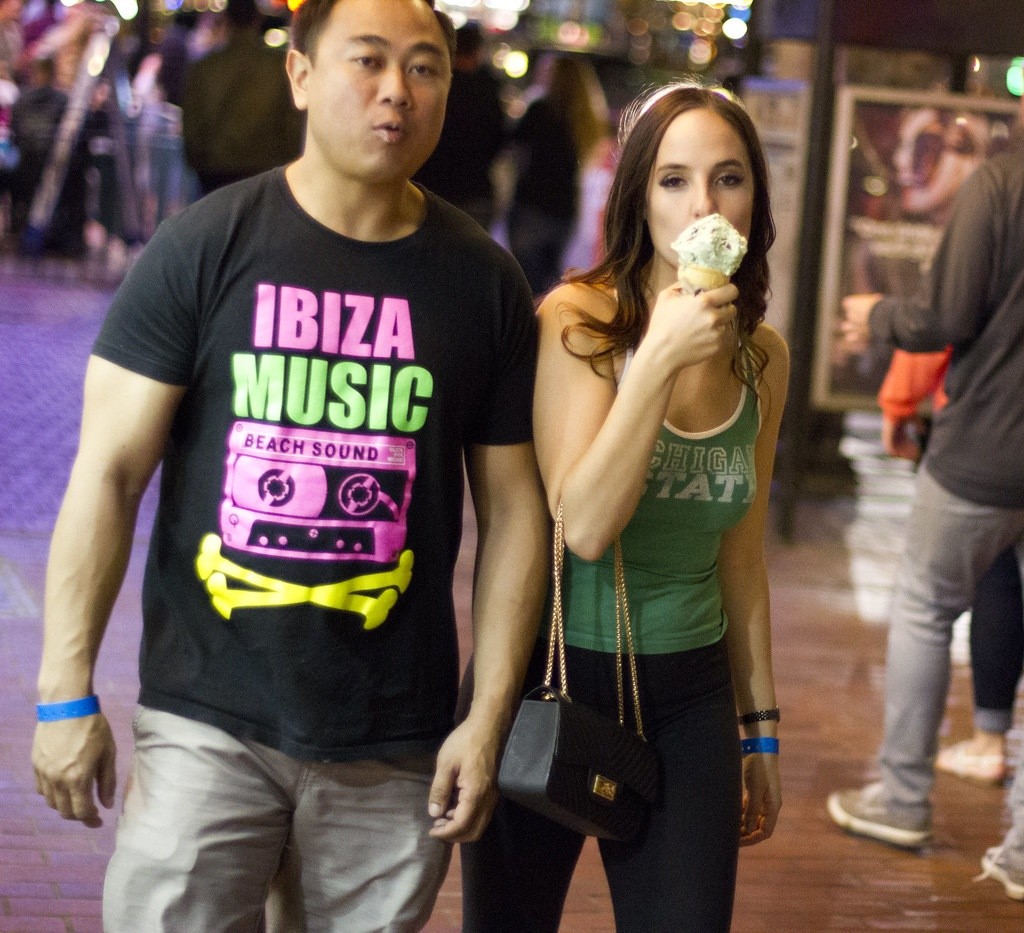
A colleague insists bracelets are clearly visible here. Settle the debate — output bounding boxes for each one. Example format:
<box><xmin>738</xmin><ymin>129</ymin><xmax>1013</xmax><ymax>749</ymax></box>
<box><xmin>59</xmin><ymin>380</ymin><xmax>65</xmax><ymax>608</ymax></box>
<box><xmin>740</xmin><ymin>705</ymin><xmax>780</xmax><ymax>723</ymax></box>
<box><xmin>741</xmin><ymin>737</ymin><xmax>780</xmax><ymax>754</ymax></box>
<box><xmin>35</xmin><ymin>693</ymin><xmax>101</xmax><ymax>722</ymax></box>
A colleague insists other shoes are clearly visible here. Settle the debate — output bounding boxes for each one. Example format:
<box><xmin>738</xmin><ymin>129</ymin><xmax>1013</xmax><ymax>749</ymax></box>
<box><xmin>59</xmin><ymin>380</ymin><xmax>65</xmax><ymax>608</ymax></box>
<box><xmin>934</xmin><ymin>740</ymin><xmax>1008</xmax><ymax>787</ymax></box>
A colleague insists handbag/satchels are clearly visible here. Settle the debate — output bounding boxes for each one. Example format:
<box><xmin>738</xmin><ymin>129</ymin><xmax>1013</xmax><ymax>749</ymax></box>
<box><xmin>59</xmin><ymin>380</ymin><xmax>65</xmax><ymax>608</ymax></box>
<box><xmin>497</xmin><ymin>497</ymin><xmax>684</xmax><ymax>843</ymax></box>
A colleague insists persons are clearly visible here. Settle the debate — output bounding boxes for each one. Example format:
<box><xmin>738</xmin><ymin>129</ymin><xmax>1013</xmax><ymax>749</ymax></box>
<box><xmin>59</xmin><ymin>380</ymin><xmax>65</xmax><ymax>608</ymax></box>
<box><xmin>31</xmin><ymin>1</ymin><xmax>552</xmax><ymax>933</ymax></box>
<box><xmin>845</xmin><ymin>71</ymin><xmax>1010</xmax><ymax>293</ymax></box>
<box><xmin>455</xmin><ymin>88</ymin><xmax>789</xmax><ymax>933</ymax></box>
<box><xmin>875</xmin><ymin>346</ymin><xmax>1024</xmax><ymax>781</ymax></box>
<box><xmin>0</xmin><ymin>0</ymin><xmax>309</xmax><ymax>282</ymax></box>
<box><xmin>827</xmin><ymin>97</ymin><xmax>1024</xmax><ymax>900</ymax></box>
<box><xmin>410</xmin><ymin>20</ymin><xmax>610</xmax><ymax>296</ymax></box>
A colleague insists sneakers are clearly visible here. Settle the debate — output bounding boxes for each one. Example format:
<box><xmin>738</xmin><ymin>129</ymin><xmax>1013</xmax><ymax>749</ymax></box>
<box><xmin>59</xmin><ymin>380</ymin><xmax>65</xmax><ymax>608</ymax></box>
<box><xmin>827</xmin><ymin>782</ymin><xmax>934</xmax><ymax>849</ymax></box>
<box><xmin>972</xmin><ymin>846</ymin><xmax>1024</xmax><ymax>900</ymax></box>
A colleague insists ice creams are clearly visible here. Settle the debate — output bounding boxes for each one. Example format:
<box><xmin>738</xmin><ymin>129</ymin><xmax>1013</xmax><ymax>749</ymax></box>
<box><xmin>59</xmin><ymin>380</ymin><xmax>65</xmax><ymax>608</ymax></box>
<box><xmin>672</xmin><ymin>213</ymin><xmax>748</xmax><ymax>295</ymax></box>
